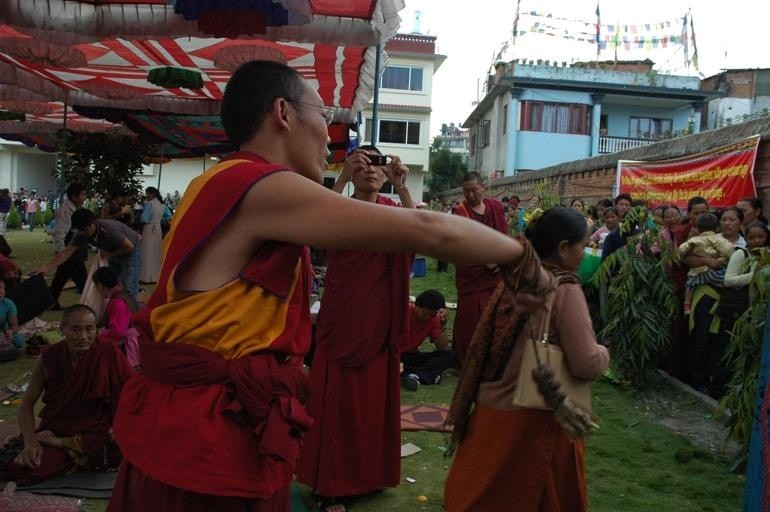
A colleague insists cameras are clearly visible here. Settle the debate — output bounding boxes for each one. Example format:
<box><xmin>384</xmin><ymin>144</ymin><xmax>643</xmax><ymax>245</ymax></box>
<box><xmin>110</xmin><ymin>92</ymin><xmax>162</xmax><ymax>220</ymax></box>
<box><xmin>365</xmin><ymin>155</ymin><xmax>387</xmax><ymax>166</ymax></box>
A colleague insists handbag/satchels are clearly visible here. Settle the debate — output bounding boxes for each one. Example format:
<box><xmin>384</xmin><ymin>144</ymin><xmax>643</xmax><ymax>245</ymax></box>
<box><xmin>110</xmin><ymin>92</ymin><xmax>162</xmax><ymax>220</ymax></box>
<box><xmin>510</xmin><ymin>336</ymin><xmax>592</xmax><ymax>415</ymax></box>
<box><xmin>715</xmin><ymin>282</ymin><xmax>754</xmax><ymax>321</ymax></box>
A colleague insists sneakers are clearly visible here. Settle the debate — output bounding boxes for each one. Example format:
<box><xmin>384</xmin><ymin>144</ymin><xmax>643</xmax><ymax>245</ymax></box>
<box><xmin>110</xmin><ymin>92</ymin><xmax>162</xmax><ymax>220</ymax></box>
<box><xmin>399</xmin><ymin>372</ymin><xmax>420</xmax><ymax>393</ymax></box>
<box><xmin>422</xmin><ymin>372</ymin><xmax>442</xmax><ymax>385</ymax></box>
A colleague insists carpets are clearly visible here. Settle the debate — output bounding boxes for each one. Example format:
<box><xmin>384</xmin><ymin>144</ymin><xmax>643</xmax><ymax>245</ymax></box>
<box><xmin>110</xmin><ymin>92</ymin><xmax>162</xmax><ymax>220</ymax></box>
<box><xmin>0</xmin><ymin>418</ymin><xmax>124</xmax><ymax>512</ymax></box>
<box><xmin>399</xmin><ymin>403</ymin><xmax>458</xmax><ymax>432</ymax></box>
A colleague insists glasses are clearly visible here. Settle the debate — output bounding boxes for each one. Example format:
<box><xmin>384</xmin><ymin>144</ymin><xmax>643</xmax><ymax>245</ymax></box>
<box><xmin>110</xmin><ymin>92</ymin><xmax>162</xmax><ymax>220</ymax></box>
<box><xmin>286</xmin><ymin>97</ymin><xmax>335</xmax><ymax>126</ymax></box>
